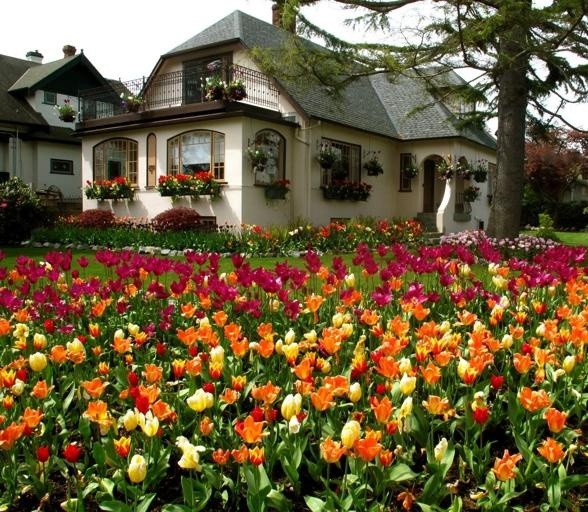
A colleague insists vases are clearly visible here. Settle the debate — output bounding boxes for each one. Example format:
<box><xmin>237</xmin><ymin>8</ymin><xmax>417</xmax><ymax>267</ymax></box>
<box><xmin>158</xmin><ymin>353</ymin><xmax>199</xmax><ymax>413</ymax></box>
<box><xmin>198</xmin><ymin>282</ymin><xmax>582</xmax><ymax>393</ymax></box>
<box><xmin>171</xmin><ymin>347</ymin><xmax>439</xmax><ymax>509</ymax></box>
<box><xmin>62</xmin><ymin>113</ymin><xmax>75</xmax><ymax>122</ymax></box>
<box><xmin>128</xmin><ymin>103</ymin><xmax>140</xmax><ymax>113</ymax></box>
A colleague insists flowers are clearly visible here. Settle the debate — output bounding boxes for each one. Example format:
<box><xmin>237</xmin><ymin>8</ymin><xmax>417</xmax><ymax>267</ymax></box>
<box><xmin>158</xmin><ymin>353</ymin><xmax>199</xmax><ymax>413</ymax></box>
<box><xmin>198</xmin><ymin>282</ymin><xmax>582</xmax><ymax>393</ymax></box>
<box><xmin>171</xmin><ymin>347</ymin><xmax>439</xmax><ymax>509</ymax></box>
<box><xmin>243</xmin><ymin>140</ymin><xmax>489</xmax><ymax>202</ymax></box>
<box><xmin>81</xmin><ymin>170</ymin><xmax>224</xmax><ymax>204</ymax></box>
<box><xmin>121</xmin><ymin>94</ymin><xmax>146</xmax><ymax>108</ymax></box>
<box><xmin>202</xmin><ymin>73</ymin><xmax>249</xmax><ymax>104</ymax></box>
<box><xmin>56</xmin><ymin>98</ymin><xmax>78</xmax><ymax>117</ymax></box>
